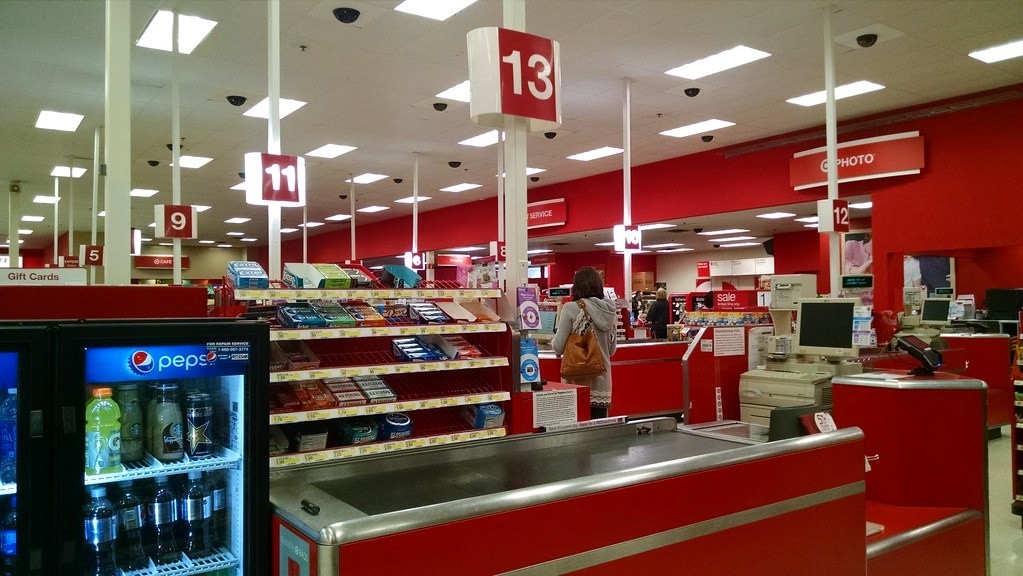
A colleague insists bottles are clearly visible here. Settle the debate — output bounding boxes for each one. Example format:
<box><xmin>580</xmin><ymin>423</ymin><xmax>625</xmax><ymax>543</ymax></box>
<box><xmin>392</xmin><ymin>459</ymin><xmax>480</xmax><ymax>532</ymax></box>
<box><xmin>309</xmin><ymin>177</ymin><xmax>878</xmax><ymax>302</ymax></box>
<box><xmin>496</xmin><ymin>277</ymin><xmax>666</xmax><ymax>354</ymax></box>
<box><xmin>147</xmin><ymin>386</ymin><xmax>158</xmax><ymax>453</ymax></box>
<box><xmin>85</xmin><ymin>387</ymin><xmax>122</xmax><ymax>475</ymax></box>
<box><xmin>0</xmin><ymin>496</ymin><xmax>17</xmax><ymax>575</ymax></box>
<box><xmin>118</xmin><ymin>385</ymin><xmax>144</xmax><ymax>462</ymax></box>
<box><xmin>153</xmin><ymin>383</ymin><xmax>184</xmax><ymax>462</ymax></box>
<box><xmin>113</xmin><ymin>480</ymin><xmax>147</xmax><ymax>571</ymax></box>
<box><xmin>82</xmin><ymin>484</ymin><xmax>119</xmax><ymax>576</ymax></box>
<box><xmin>148</xmin><ymin>475</ymin><xmax>180</xmax><ymax>565</ymax></box>
<box><xmin>0</xmin><ymin>388</ymin><xmax>16</xmax><ymax>483</ymax></box>
<box><xmin>210</xmin><ymin>469</ymin><xmax>226</xmax><ymax>548</ymax></box>
<box><xmin>173</xmin><ymin>471</ymin><xmax>211</xmax><ymax>559</ymax></box>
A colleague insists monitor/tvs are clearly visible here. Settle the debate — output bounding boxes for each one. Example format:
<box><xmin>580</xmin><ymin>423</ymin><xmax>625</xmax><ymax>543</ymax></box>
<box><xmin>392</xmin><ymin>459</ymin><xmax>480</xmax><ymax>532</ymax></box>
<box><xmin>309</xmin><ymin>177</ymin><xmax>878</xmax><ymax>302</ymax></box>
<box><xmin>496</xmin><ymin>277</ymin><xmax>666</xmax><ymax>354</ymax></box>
<box><xmin>792</xmin><ymin>297</ymin><xmax>861</xmax><ymax>362</ymax></box>
<box><xmin>920</xmin><ymin>298</ymin><xmax>953</xmax><ymax>330</ymax></box>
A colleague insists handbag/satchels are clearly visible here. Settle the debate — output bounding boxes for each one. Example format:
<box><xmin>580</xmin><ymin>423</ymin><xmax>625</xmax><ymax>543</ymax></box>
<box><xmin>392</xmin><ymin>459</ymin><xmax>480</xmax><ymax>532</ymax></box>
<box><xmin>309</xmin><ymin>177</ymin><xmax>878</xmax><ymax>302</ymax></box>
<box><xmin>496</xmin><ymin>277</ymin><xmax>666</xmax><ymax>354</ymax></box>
<box><xmin>561</xmin><ymin>301</ymin><xmax>608</xmax><ymax>379</ymax></box>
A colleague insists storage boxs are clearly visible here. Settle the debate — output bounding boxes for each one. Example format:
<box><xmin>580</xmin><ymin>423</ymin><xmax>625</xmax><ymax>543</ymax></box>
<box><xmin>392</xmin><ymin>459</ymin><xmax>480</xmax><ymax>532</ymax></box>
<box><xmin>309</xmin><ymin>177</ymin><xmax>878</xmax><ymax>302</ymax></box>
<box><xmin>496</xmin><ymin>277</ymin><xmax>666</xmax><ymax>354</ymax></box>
<box><xmin>459</xmin><ymin>301</ymin><xmax>502</xmax><ymax>324</ymax></box>
<box><xmin>282</xmin><ymin>263</ymin><xmax>326</xmax><ymax>289</ymax></box>
<box><xmin>432</xmin><ymin>301</ymin><xmax>479</xmax><ymax>325</ymax></box>
<box><xmin>309</xmin><ymin>303</ymin><xmax>357</xmax><ymax>328</ymax></box>
<box><xmin>338</xmin><ymin>264</ymin><xmax>382</xmax><ymax>289</ymax></box>
<box><xmin>227</xmin><ymin>260</ymin><xmax>270</xmax><ymax>289</ymax></box>
<box><xmin>276</xmin><ymin>302</ymin><xmax>326</xmax><ymax>329</ymax></box>
<box><xmin>274</xmin><ymin>340</ymin><xmax>321</xmax><ymax>371</ymax></box>
<box><xmin>269</xmin><ymin>341</ymin><xmax>288</xmax><ymax>372</ymax></box>
<box><xmin>270</xmin><ymin>382</ymin><xmax>303</xmax><ymax>414</ymax></box>
<box><xmin>339</xmin><ymin>302</ymin><xmax>386</xmax><ymax>328</ymax></box>
<box><xmin>380</xmin><ymin>264</ymin><xmax>422</xmax><ymax>289</ymax></box>
<box><xmin>289</xmin><ymin>380</ymin><xmax>338</xmax><ymax>412</ymax></box>
<box><xmin>334</xmin><ymin>416</ymin><xmax>378</xmax><ymax>445</ymax></box>
<box><xmin>406</xmin><ymin>302</ymin><xmax>453</xmax><ymax>324</ymax></box>
<box><xmin>371</xmin><ymin>412</ymin><xmax>414</xmax><ymax>442</ymax></box>
<box><xmin>459</xmin><ymin>402</ymin><xmax>505</xmax><ymax>429</ymax></box>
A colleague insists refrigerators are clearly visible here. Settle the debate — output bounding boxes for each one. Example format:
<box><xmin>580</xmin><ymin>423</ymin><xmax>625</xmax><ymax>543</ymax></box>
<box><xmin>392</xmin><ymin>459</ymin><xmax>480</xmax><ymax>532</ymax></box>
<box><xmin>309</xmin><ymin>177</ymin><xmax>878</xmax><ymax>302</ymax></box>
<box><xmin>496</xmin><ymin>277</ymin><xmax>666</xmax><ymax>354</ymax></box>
<box><xmin>0</xmin><ymin>321</ymin><xmax>272</xmax><ymax>576</ymax></box>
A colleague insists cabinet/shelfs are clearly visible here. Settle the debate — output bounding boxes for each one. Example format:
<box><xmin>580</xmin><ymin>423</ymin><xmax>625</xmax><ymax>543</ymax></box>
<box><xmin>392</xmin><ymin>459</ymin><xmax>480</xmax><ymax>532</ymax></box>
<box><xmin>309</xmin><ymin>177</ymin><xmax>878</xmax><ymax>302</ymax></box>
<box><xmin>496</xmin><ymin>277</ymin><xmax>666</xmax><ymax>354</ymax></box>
<box><xmin>223</xmin><ymin>277</ymin><xmax>517</xmax><ymax>470</ymax></box>
<box><xmin>1009</xmin><ymin>309</ymin><xmax>1023</xmax><ymax>530</ymax></box>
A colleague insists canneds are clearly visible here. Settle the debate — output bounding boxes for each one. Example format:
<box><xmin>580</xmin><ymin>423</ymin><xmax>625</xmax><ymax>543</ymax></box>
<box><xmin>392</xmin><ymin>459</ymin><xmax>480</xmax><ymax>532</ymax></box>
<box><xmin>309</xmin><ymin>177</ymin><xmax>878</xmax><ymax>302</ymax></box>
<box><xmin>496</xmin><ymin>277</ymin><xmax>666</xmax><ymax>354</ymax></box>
<box><xmin>181</xmin><ymin>390</ymin><xmax>214</xmax><ymax>458</ymax></box>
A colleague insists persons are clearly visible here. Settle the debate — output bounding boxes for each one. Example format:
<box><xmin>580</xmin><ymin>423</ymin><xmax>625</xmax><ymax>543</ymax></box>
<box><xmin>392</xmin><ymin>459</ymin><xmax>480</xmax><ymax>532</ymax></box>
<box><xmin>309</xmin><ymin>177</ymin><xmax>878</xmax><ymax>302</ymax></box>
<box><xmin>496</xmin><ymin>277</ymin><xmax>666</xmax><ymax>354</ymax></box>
<box><xmin>646</xmin><ymin>287</ymin><xmax>674</xmax><ymax>339</ymax></box>
<box><xmin>632</xmin><ymin>290</ymin><xmax>647</xmax><ymax>321</ymax></box>
<box><xmin>551</xmin><ymin>266</ymin><xmax>618</xmax><ymax>419</ymax></box>
<box><xmin>844</xmin><ymin>239</ymin><xmax>872</xmax><ymax>275</ymax></box>
<box><xmin>904</xmin><ymin>256</ymin><xmax>950</xmax><ymax>297</ymax></box>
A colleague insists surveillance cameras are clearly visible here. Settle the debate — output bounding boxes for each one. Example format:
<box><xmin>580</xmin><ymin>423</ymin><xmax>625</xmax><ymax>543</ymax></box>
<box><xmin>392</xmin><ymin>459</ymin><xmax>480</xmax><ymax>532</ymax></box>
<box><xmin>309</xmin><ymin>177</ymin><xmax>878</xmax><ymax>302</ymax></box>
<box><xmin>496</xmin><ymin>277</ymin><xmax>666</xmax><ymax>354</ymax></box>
<box><xmin>856</xmin><ymin>33</ymin><xmax>878</xmax><ymax>48</ymax></box>
<box><xmin>393</xmin><ymin>179</ymin><xmax>403</xmax><ymax>184</ymax></box>
<box><xmin>147</xmin><ymin>160</ymin><xmax>160</xmax><ymax>166</ymax></box>
<box><xmin>332</xmin><ymin>8</ymin><xmax>360</xmax><ymax>24</ymax></box>
<box><xmin>544</xmin><ymin>131</ymin><xmax>556</xmax><ymax>140</ymax></box>
<box><xmin>684</xmin><ymin>88</ymin><xmax>700</xmax><ymax>98</ymax></box>
<box><xmin>432</xmin><ymin>103</ymin><xmax>447</xmax><ymax>112</ymax></box>
<box><xmin>226</xmin><ymin>96</ymin><xmax>247</xmax><ymax>107</ymax></box>
<box><xmin>448</xmin><ymin>162</ymin><xmax>461</xmax><ymax>168</ymax></box>
<box><xmin>701</xmin><ymin>136</ymin><xmax>714</xmax><ymax>142</ymax></box>
<box><xmin>166</xmin><ymin>144</ymin><xmax>184</xmax><ymax>151</ymax></box>
<box><xmin>530</xmin><ymin>177</ymin><xmax>539</xmax><ymax>182</ymax></box>
<box><xmin>339</xmin><ymin>195</ymin><xmax>347</xmax><ymax>199</ymax></box>
